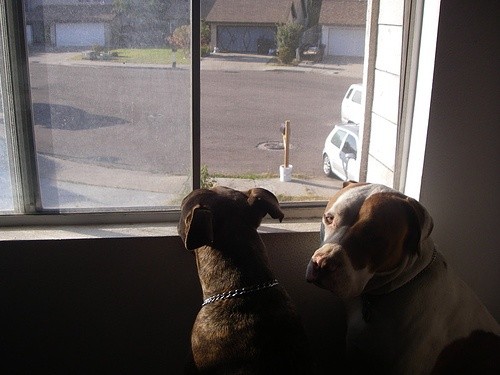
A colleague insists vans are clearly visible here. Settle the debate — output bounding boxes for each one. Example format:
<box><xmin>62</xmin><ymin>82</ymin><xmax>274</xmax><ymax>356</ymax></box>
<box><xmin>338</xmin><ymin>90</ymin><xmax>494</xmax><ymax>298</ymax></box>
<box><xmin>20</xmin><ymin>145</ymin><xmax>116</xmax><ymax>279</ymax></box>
<box><xmin>342</xmin><ymin>84</ymin><xmax>362</xmax><ymax>124</ymax></box>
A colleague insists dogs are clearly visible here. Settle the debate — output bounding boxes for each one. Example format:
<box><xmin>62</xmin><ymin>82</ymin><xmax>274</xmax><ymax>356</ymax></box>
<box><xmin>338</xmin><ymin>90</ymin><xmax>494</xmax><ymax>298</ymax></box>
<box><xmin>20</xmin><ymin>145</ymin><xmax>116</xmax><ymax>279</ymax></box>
<box><xmin>177</xmin><ymin>186</ymin><xmax>346</xmax><ymax>374</ymax></box>
<box><xmin>305</xmin><ymin>180</ymin><xmax>499</xmax><ymax>374</ymax></box>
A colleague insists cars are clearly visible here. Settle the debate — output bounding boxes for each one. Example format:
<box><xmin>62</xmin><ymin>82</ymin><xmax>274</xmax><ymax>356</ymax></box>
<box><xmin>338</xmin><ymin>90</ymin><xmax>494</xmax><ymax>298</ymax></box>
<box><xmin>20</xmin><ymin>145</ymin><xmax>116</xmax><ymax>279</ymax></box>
<box><xmin>322</xmin><ymin>125</ymin><xmax>360</xmax><ymax>183</ymax></box>
